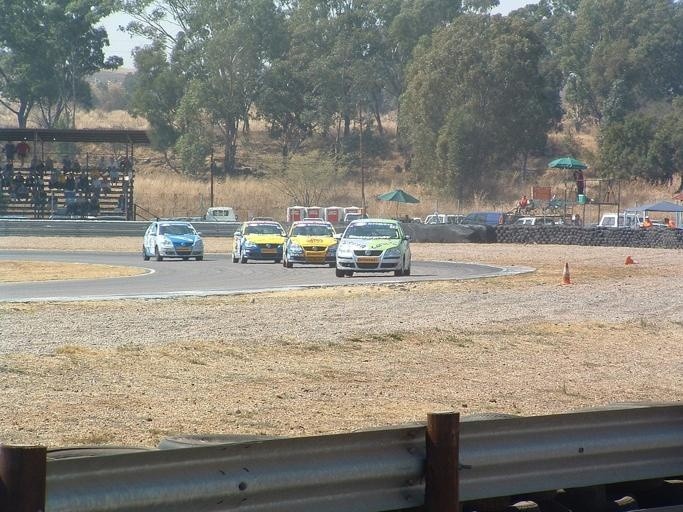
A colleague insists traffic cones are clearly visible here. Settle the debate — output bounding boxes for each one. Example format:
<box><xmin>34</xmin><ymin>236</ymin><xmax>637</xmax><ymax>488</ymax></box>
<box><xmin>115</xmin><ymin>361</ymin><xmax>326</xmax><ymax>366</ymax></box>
<box><xmin>561</xmin><ymin>261</ymin><xmax>571</xmax><ymax>284</ymax></box>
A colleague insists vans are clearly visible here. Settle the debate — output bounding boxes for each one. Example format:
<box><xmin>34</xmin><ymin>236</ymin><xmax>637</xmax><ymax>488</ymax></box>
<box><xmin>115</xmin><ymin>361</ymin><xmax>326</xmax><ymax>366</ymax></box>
<box><xmin>344</xmin><ymin>213</ymin><xmax>369</xmax><ymax>222</ymax></box>
<box><xmin>412</xmin><ymin>213</ymin><xmax>672</xmax><ymax>229</ymax></box>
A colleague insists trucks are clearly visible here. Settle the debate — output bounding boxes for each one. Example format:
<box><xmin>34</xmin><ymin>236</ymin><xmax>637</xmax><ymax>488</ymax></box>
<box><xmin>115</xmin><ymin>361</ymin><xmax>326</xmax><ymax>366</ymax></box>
<box><xmin>203</xmin><ymin>206</ymin><xmax>237</xmax><ymax>222</ymax></box>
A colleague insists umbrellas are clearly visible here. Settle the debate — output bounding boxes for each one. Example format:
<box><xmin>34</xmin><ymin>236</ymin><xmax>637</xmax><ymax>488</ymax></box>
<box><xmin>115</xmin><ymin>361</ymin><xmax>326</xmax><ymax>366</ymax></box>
<box><xmin>545</xmin><ymin>156</ymin><xmax>588</xmax><ymax>191</ymax></box>
<box><xmin>376</xmin><ymin>188</ymin><xmax>420</xmax><ymax>222</ymax></box>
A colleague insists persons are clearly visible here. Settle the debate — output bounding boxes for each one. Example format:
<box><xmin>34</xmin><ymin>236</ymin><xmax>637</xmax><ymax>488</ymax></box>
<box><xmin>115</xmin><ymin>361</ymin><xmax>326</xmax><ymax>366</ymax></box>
<box><xmin>0</xmin><ymin>139</ymin><xmax>134</xmax><ymax>221</ymax></box>
<box><xmin>442</xmin><ymin>213</ymin><xmax>448</xmax><ymax>223</ymax></box>
<box><xmin>663</xmin><ymin>217</ymin><xmax>675</xmax><ymax>227</ymax></box>
<box><xmin>510</xmin><ymin>193</ymin><xmax>527</xmax><ymax>211</ymax></box>
<box><xmin>524</xmin><ymin>197</ymin><xmax>535</xmax><ymax>213</ymax></box>
<box><xmin>403</xmin><ymin>214</ymin><xmax>410</xmax><ymax>219</ymax></box>
<box><xmin>573</xmin><ymin>212</ymin><xmax>583</xmax><ymax>228</ymax></box>
<box><xmin>640</xmin><ymin>215</ymin><xmax>652</xmax><ymax>229</ymax></box>
<box><xmin>543</xmin><ymin>193</ymin><xmax>562</xmax><ymax>213</ymax></box>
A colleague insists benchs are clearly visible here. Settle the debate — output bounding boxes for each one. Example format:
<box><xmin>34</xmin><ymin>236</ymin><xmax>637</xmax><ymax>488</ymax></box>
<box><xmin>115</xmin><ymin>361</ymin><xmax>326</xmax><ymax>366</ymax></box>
<box><xmin>0</xmin><ymin>149</ymin><xmax>134</xmax><ymax>225</ymax></box>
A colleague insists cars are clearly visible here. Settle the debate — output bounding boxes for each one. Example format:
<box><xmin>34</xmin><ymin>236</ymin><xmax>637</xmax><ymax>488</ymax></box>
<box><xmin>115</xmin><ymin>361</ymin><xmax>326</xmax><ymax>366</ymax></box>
<box><xmin>230</xmin><ymin>217</ymin><xmax>341</xmax><ymax>268</ymax></box>
<box><xmin>140</xmin><ymin>217</ymin><xmax>205</xmax><ymax>264</ymax></box>
<box><xmin>332</xmin><ymin>217</ymin><xmax>413</xmax><ymax>278</ymax></box>
